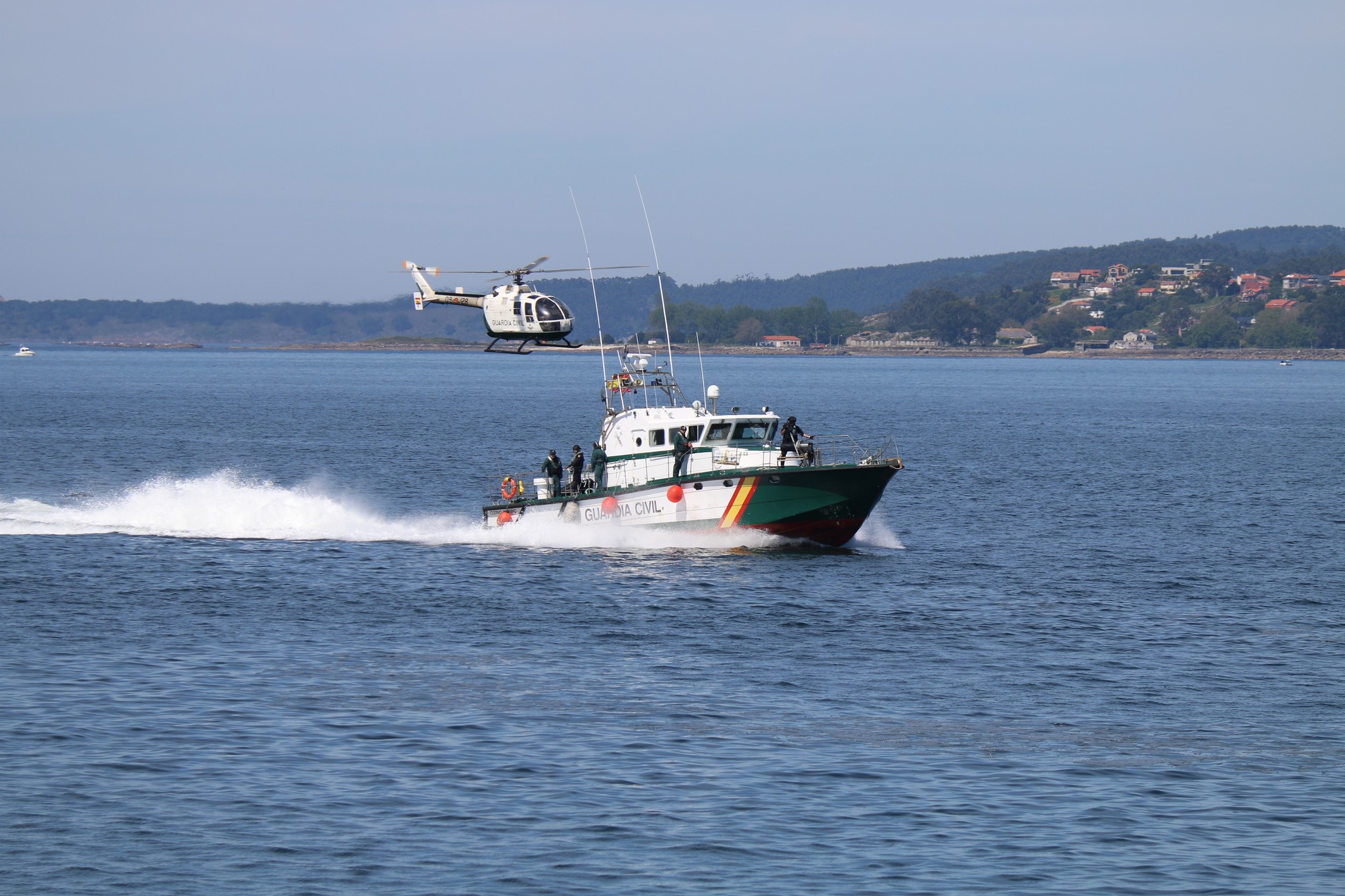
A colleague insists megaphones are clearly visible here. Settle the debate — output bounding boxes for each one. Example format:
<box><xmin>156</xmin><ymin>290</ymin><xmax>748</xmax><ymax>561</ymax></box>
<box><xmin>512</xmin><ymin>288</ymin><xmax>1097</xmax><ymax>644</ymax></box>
<box><xmin>634</xmin><ymin>379</ymin><xmax>643</xmax><ymax>385</ymax></box>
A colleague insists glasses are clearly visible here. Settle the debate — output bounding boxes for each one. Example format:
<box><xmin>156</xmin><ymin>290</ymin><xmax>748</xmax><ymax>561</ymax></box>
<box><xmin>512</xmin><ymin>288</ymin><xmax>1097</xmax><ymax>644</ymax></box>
<box><xmin>551</xmin><ymin>454</ymin><xmax>556</xmax><ymax>456</ymax></box>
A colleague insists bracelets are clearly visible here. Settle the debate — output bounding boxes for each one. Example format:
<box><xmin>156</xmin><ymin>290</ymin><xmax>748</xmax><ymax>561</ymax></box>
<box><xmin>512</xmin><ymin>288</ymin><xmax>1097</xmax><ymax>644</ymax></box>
<box><xmin>809</xmin><ymin>436</ymin><xmax>810</xmax><ymax>438</ymax></box>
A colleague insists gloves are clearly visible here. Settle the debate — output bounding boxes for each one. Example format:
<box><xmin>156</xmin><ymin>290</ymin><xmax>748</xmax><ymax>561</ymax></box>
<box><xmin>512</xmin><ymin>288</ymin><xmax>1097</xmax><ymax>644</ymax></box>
<box><xmin>542</xmin><ymin>469</ymin><xmax>545</xmax><ymax>472</ymax></box>
<box><xmin>592</xmin><ymin>469</ymin><xmax>595</xmax><ymax>473</ymax></box>
<box><xmin>564</xmin><ymin>466</ymin><xmax>569</xmax><ymax>471</ymax></box>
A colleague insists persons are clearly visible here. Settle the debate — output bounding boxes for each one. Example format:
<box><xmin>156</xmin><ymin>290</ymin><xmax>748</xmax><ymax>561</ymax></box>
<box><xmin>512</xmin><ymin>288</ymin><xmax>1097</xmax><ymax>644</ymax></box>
<box><xmin>549</xmin><ymin>299</ymin><xmax>554</xmax><ymax>307</ymax></box>
<box><xmin>536</xmin><ymin>300</ymin><xmax>546</xmax><ymax>321</ymax></box>
<box><xmin>591</xmin><ymin>442</ymin><xmax>609</xmax><ymax>493</ymax></box>
<box><xmin>780</xmin><ymin>416</ymin><xmax>816</xmax><ymax>468</ymax></box>
<box><xmin>565</xmin><ymin>445</ymin><xmax>585</xmax><ymax>495</ymax></box>
<box><xmin>542</xmin><ymin>450</ymin><xmax>564</xmax><ymax>498</ymax></box>
<box><xmin>673</xmin><ymin>426</ymin><xmax>692</xmax><ymax>479</ymax></box>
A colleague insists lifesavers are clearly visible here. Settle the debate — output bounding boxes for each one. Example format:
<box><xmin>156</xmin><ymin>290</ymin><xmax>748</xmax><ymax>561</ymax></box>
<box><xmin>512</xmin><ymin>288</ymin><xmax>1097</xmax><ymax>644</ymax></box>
<box><xmin>501</xmin><ymin>478</ymin><xmax>516</xmax><ymax>499</ymax></box>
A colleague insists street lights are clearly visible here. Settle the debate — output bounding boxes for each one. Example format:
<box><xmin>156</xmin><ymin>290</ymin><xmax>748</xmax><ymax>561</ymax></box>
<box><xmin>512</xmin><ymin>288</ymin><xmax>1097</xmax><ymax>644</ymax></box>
<box><xmin>838</xmin><ymin>335</ymin><xmax>842</xmax><ymax>346</ymax></box>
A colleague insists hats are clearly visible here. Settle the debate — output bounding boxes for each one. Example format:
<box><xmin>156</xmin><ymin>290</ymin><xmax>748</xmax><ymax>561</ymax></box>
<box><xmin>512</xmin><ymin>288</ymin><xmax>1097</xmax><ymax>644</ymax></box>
<box><xmin>787</xmin><ymin>416</ymin><xmax>797</xmax><ymax>422</ymax></box>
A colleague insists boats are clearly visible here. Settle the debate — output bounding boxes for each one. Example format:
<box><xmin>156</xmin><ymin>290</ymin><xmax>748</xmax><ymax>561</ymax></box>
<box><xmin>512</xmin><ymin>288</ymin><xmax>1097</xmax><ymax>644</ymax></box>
<box><xmin>15</xmin><ymin>344</ymin><xmax>35</xmax><ymax>356</ymax></box>
<box><xmin>483</xmin><ymin>164</ymin><xmax>905</xmax><ymax>554</ymax></box>
<box><xmin>1279</xmin><ymin>359</ymin><xmax>1293</xmax><ymax>366</ymax></box>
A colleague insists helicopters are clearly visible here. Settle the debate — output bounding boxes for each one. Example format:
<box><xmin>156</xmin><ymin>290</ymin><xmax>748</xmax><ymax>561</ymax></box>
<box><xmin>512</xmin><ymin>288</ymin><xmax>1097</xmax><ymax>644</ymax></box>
<box><xmin>388</xmin><ymin>255</ymin><xmax>650</xmax><ymax>355</ymax></box>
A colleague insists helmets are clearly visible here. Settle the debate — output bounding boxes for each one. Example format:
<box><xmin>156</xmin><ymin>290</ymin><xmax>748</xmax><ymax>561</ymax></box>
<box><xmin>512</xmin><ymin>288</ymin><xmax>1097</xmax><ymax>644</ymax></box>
<box><xmin>573</xmin><ymin>445</ymin><xmax>581</xmax><ymax>450</ymax></box>
<box><xmin>592</xmin><ymin>441</ymin><xmax>599</xmax><ymax>447</ymax></box>
<box><xmin>550</xmin><ymin>450</ymin><xmax>557</xmax><ymax>454</ymax></box>
<box><xmin>680</xmin><ymin>426</ymin><xmax>687</xmax><ymax>430</ymax></box>
